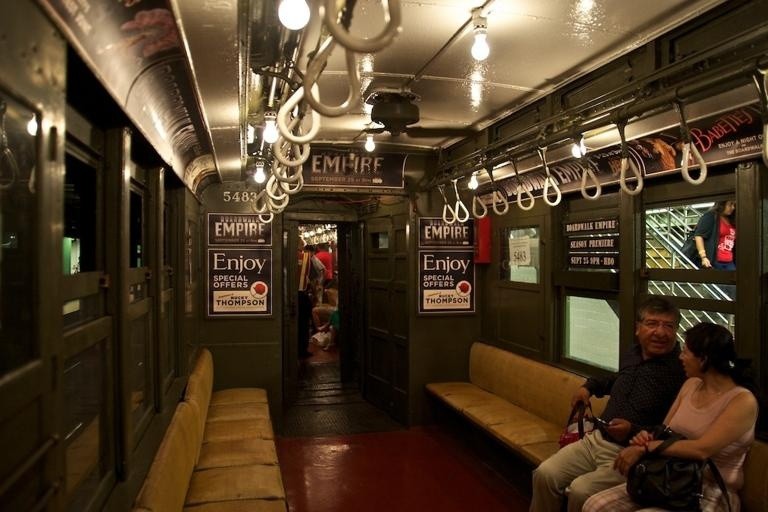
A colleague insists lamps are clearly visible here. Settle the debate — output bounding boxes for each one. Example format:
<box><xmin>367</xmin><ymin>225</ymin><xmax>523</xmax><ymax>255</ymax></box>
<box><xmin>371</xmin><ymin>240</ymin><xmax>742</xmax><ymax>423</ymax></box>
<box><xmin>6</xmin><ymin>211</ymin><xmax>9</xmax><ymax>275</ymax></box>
<box><xmin>467</xmin><ymin>169</ymin><xmax>480</xmax><ymax>189</ymax></box>
<box><xmin>471</xmin><ymin>16</ymin><xmax>490</xmax><ymax>62</ymax></box>
<box><xmin>360</xmin><ymin>55</ymin><xmax>378</xmax><ymax>154</ymax></box>
<box><xmin>572</xmin><ymin>133</ymin><xmax>588</xmax><ymax>160</ymax></box>
<box><xmin>254</xmin><ymin>0</ymin><xmax>311</xmax><ymax>190</ymax></box>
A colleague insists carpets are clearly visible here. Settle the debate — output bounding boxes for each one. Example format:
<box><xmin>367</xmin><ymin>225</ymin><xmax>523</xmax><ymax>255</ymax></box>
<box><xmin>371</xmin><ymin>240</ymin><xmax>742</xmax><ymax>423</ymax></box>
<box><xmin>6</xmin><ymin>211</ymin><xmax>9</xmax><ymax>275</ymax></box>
<box><xmin>277</xmin><ymin>427</ymin><xmax>533</xmax><ymax>511</ymax></box>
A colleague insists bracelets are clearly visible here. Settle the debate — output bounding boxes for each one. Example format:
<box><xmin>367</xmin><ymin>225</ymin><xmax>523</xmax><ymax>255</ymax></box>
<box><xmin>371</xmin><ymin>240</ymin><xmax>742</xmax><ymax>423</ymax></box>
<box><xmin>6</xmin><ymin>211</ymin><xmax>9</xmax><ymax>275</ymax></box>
<box><xmin>700</xmin><ymin>250</ymin><xmax>705</xmax><ymax>254</ymax></box>
<box><xmin>701</xmin><ymin>256</ymin><xmax>706</xmax><ymax>260</ymax></box>
<box><xmin>643</xmin><ymin>442</ymin><xmax>649</xmax><ymax>455</ymax></box>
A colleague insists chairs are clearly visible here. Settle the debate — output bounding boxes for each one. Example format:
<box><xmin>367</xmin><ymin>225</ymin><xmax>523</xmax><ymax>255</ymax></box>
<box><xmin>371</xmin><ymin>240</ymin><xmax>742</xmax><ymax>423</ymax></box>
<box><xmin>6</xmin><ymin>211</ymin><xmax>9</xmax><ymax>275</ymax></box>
<box><xmin>424</xmin><ymin>342</ymin><xmax>768</xmax><ymax>512</ymax></box>
<box><xmin>100</xmin><ymin>342</ymin><xmax>288</xmax><ymax>511</ymax></box>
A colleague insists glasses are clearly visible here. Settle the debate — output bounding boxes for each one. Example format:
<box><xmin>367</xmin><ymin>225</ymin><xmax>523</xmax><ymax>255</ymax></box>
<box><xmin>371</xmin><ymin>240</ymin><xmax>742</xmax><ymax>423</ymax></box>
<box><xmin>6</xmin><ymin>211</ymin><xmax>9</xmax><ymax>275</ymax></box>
<box><xmin>638</xmin><ymin>320</ymin><xmax>678</xmax><ymax>332</ymax></box>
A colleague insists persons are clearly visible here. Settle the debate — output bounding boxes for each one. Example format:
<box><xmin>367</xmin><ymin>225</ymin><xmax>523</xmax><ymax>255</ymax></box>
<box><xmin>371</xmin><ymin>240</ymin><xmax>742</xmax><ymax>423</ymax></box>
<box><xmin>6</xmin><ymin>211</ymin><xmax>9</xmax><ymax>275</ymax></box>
<box><xmin>580</xmin><ymin>321</ymin><xmax>760</xmax><ymax>511</ymax></box>
<box><xmin>689</xmin><ymin>196</ymin><xmax>736</xmax><ymax>324</ymax></box>
<box><xmin>296</xmin><ymin>235</ymin><xmax>338</xmax><ymax>360</ymax></box>
<box><xmin>528</xmin><ymin>295</ymin><xmax>688</xmax><ymax>510</ymax></box>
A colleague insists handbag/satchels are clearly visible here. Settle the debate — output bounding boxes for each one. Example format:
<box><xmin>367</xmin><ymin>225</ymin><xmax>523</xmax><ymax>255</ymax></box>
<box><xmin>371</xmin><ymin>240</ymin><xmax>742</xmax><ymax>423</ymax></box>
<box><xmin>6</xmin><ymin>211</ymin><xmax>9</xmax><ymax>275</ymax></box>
<box><xmin>680</xmin><ymin>230</ymin><xmax>698</xmax><ymax>264</ymax></box>
<box><xmin>558</xmin><ymin>400</ymin><xmax>598</xmax><ymax>451</ymax></box>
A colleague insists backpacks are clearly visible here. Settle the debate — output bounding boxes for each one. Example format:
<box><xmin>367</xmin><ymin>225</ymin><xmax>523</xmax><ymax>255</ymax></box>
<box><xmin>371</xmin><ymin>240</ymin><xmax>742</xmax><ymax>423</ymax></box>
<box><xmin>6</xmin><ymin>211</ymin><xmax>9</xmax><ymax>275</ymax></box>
<box><xmin>626</xmin><ymin>426</ymin><xmax>732</xmax><ymax>512</ymax></box>
